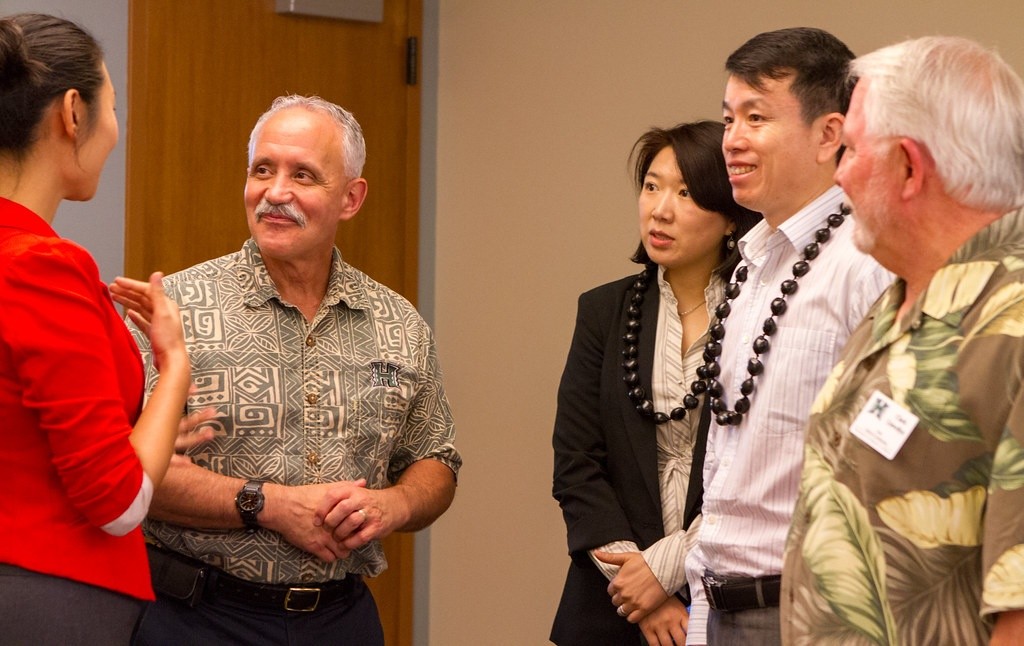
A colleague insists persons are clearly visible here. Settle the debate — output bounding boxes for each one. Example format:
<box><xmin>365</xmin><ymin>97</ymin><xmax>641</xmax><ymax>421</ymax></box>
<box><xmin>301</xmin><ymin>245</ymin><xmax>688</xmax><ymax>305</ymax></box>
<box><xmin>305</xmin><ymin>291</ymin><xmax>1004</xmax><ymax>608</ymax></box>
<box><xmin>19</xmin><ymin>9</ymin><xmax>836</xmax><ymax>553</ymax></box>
<box><xmin>684</xmin><ymin>25</ymin><xmax>897</xmax><ymax>646</ymax></box>
<box><xmin>123</xmin><ymin>96</ymin><xmax>462</xmax><ymax>646</ymax></box>
<box><xmin>0</xmin><ymin>13</ymin><xmax>192</xmax><ymax>645</ymax></box>
<box><xmin>549</xmin><ymin>120</ymin><xmax>763</xmax><ymax>646</ymax></box>
<box><xmin>780</xmin><ymin>34</ymin><xmax>1024</xmax><ymax>646</ymax></box>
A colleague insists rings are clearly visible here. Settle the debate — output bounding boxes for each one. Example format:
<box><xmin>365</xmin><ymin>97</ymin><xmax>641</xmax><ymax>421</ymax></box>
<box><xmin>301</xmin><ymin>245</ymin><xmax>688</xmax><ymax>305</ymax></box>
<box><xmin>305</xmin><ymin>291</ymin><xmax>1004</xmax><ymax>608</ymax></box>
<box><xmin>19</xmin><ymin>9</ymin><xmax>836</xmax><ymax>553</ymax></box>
<box><xmin>359</xmin><ymin>509</ymin><xmax>369</xmax><ymax>522</ymax></box>
<box><xmin>620</xmin><ymin>606</ymin><xmax>628</xmax><ymax>616</ymax></box>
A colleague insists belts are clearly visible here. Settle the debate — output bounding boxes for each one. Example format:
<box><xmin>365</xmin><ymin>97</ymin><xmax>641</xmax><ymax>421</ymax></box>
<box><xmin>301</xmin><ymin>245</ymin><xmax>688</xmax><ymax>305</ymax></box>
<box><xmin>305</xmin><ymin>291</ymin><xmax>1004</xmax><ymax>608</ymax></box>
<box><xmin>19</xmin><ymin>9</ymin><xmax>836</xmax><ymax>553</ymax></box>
<box><xmin>701</xmin><ymin>576</ymin><xmax>781</xmax><ymax>613</ymax></box>
<box><xmin>202</xmin><ymin>567</ymin><xmax>362</xmax><ymax>613</ymax></box>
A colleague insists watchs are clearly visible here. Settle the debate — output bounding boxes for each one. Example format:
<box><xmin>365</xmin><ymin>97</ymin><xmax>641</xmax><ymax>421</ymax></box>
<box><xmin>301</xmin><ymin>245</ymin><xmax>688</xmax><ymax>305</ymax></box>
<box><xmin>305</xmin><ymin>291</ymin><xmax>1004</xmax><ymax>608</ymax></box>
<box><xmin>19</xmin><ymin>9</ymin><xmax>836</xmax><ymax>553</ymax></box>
<box><xmin>235</xmin><ymin>480</ymin><xmax>266</xmax><ymax>528</ymax></box>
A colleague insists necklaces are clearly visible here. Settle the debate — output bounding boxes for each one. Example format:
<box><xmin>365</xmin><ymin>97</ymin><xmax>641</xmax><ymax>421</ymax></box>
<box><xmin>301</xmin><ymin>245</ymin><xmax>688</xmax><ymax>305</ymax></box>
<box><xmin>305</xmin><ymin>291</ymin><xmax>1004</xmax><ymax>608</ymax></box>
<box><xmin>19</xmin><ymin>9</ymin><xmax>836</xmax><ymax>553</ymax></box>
<box><xmin>621</xmin><ymin>269</ymin><xmax>714</xmax><ymax>424</ymax></box>
<box><xmin>706</xmin><ymin>203</ymin><xmax>853</xmax><ymax>426</ymax></box>
